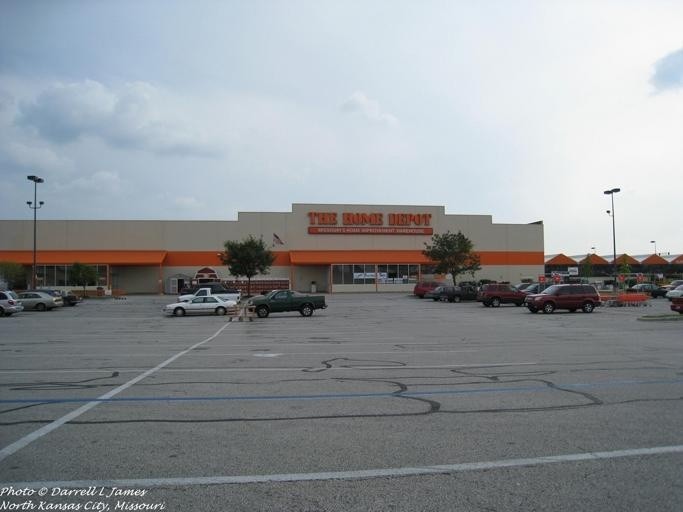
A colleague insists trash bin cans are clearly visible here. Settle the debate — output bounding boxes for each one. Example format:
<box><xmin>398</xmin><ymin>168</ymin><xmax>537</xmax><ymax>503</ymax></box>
<box><xmin>310</xmin><ymin>280</ymin><xmax>317</xmax><ymax>293</ymax></box>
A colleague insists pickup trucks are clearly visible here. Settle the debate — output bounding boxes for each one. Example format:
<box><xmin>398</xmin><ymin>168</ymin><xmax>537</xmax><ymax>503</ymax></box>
<box><xmin>247</xmin><ymin>289</ymin><xmax>328</xmax><ymax>317</ymax></box>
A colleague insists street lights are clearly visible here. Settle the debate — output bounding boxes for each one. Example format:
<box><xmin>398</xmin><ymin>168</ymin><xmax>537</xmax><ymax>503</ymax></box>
<box><xmin>650</xmin><ymin>240</ymin><xmax>657</xmax><ymax>255</ymax></box>
<box><xmin>25</xmin><ymin>174</ymin><xmax>46</xmax><ymax>293</ymax></box>
<box><xmin>603</xmin><ymin>188</ymin><xmax>621</xmax><ymax>295</ymax></box>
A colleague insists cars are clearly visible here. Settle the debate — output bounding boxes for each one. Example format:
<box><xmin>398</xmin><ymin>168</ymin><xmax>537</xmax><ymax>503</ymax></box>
<box><xmin>413</xmin><ymin>278</ymin><xmax>553</xmax><ymax>308</ymax></box>
<box><xmin>256</xmin><ymin>290</ymin><xmax>309</xmax><ymax>297</ymax></box>
<box><xmin>16</xmin><ymin>288</ymin><xmax>82</xmax><ymax>312</ymax></box>
<box><xmin>161</xmin><ymin>281</ymin><xmax>242</xmax><ymax>317</ymax></box>
<box><xmin>626</xmin><ymin>278</ymin><xmax>682</xmax><ymax>316</ymax></box>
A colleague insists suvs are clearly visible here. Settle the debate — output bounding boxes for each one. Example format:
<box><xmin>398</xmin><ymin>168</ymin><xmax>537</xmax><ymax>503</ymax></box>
<box><xmin>0</xmin><ymin>288</ymin><xmax>24</xmax><ymax>317</ymax></box>
<box><xmin>523</xmin><ymin>283</ymin><xmax>600</xmax><ymax>314</ymax></box>
